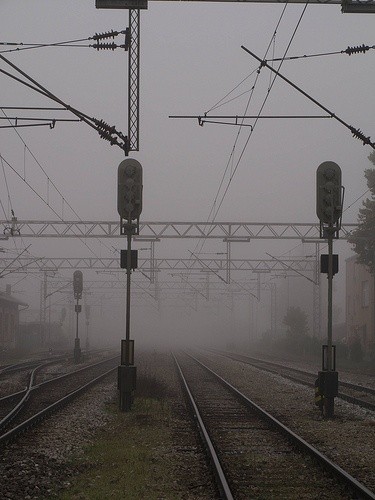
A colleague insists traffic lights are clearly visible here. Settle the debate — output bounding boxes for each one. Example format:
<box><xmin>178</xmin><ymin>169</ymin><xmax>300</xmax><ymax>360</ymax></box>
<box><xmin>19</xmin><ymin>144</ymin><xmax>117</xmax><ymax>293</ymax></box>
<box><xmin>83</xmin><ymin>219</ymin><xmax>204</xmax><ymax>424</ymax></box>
<box><xmin>73</xmin><ymin>270</ymin><xmax>84</xmax><ymax>294</ymax></box>
<box><xmin>316</xmin><ymin>161</ymin><xmax>342</xmax><ymax>225</ymax></box>
<box><xmin>117</xmin><ymin>158</ymin><xmax>144</xmax><ymax>221</ymax></box>
